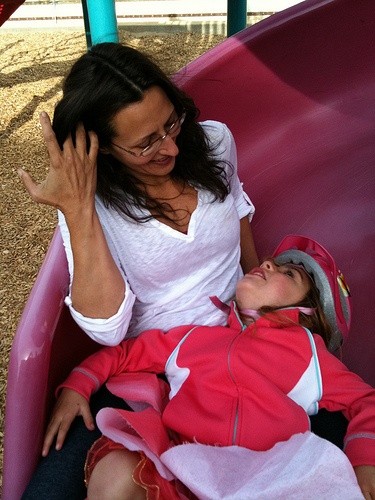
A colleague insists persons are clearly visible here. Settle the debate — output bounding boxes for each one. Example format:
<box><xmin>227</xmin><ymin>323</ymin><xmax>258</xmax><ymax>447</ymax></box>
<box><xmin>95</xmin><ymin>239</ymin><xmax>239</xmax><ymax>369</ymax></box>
<box><xmin>22</xmin><ymin>41</ymin><xmax>261</xmax><ymax>500</ymax></box>
<box><xmin>41</xmin><ymin>233</ymin><xmax>374</xmax><ymax>499</ymax></box>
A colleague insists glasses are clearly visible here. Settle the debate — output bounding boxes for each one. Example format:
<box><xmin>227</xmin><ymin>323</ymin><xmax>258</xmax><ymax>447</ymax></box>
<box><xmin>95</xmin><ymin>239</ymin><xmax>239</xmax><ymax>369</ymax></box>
<box><xmin>111</xmin><ymin>108</ymin><xmax>186</xmax><ymax>157</ymax></box>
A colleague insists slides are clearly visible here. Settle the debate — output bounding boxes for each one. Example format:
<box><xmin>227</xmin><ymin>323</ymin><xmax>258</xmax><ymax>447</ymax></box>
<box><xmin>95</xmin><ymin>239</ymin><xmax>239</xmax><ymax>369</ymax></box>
<box><xmin>1</xmin><ymin>0</ymin><xmax>375</xmax><ymax>500</ymax></box>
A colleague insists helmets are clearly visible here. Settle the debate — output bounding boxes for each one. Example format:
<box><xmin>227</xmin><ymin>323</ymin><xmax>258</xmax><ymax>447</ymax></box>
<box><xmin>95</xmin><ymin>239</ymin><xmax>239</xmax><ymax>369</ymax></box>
<box><xmin>272</xmin><ymin>234</ymin><xmax>352</xmax><ymax>354</ymax></box>
